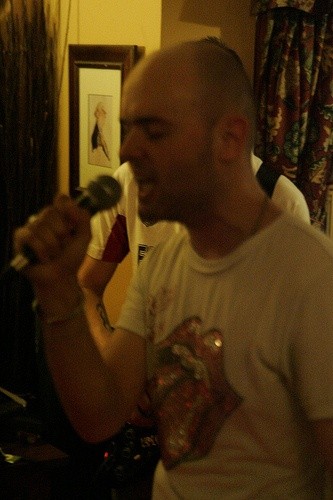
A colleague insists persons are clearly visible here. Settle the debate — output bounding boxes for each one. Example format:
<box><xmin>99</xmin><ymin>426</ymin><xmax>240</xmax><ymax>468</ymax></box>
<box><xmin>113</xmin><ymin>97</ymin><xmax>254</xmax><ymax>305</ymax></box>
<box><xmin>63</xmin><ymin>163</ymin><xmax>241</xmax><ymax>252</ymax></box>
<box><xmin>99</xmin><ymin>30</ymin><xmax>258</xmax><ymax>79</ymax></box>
<box><xmin>13</xmin><ymin>37</ymin><xmax>332</xmax><ymax>499</ymax></box>
<box><xmin>73</xmin><ymin>33</ymin><xmax>312</xmax><ymax>427</ymax></box>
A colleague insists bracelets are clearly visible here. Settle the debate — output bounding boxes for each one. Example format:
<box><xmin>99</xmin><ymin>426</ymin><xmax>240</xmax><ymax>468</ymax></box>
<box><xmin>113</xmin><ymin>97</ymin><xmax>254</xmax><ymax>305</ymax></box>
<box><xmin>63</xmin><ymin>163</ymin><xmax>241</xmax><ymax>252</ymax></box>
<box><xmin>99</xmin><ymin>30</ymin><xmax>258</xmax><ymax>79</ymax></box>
<box><xmin>30</xmin><ymin>286</ymin><xmax>85</xmax><ymax>355</ymax></box>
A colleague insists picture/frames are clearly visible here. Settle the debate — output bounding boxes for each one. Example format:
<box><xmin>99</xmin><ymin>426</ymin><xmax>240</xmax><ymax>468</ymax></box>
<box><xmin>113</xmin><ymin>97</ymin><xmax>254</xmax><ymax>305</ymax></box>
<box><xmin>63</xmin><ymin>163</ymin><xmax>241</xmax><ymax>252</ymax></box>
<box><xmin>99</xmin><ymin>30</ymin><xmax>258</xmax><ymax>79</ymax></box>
<box><xmin>68</xmin><ymin>44</ymin><xmax>138</xmax><ymax>201</ymax></box>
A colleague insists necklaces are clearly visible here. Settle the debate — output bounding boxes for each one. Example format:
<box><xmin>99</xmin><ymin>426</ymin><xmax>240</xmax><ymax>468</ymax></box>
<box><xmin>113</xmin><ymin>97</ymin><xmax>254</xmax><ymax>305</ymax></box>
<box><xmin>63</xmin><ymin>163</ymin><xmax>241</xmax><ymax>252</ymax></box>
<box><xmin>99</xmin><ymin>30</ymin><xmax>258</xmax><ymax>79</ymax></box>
<box><xmin>247</xmin><ymin>192</ymin><xmax>270</xmax><ymax>239</ymax></box>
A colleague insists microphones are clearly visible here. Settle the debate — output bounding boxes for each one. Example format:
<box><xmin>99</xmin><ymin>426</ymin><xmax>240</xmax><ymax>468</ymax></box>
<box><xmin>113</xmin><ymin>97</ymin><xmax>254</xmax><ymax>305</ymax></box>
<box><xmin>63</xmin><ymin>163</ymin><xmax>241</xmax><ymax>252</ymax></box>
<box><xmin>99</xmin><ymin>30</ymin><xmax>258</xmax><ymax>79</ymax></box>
<box><xmin>1</xmin><ymin>175</ymin><xmax>123</xmax><ymax>287</ymax></box>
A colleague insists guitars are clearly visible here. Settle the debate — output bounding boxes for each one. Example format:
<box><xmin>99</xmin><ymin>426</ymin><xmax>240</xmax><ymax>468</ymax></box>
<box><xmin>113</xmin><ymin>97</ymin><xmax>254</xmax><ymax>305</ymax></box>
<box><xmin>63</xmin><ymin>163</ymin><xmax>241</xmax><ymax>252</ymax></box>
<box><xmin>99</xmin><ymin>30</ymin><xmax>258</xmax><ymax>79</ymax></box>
<box><xmin>0</xmin><ymin>390</ymin><xmax>160</xmax><ymax>485</ymax></box>
